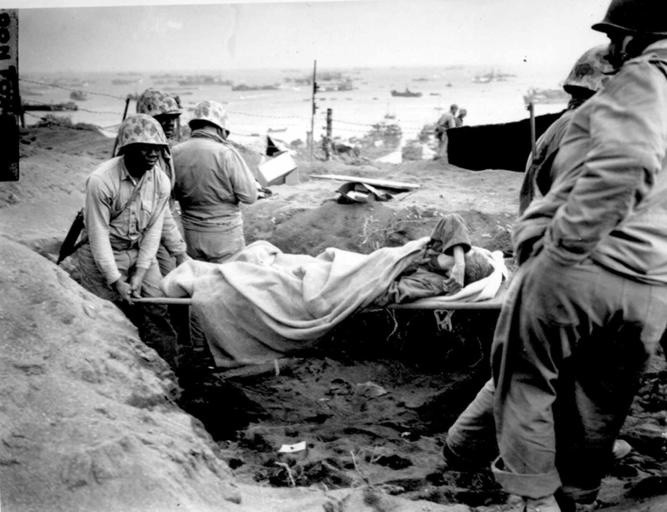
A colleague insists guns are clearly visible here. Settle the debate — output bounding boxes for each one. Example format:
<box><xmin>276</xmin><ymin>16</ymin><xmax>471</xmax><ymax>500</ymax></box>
<box><xmin>527</xmin><ymin>102</ymin><xmax>540</xmax><ymax>162</ymax></box>
<box><xmin>57</xmin><ymin>208</ymin><xmax>88</xmax><ymax>264</ymax></box>
<box><xmin>112</xmin><ymin>98</ymin><xmax>130</xmax><ymax>157</ymax></box>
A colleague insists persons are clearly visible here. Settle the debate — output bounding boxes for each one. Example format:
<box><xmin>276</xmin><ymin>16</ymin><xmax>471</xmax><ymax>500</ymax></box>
<box><xmin>439</xmin><ymin>45</ymin><xmax>621</xmax><ymax>468</ymax></box>
<box><xmin>168</xmin><ymin>99</ymin><xmax>258</xmax><ymax>354</ymax></box>
<box><xmin>75</xmin><ymin>113</ymin><xmax>179</xmax><ymax>368</ymax></box>
<box><xmin>434</xmin><ymin>104</ymin><xmax>467</xmax><ymax>160</ymax></box>
<box><xmin>368</xmin><ymin>213</ymin><xmax>495</xmax><ymax>308</ymax></box>
<box><xmin>492</xmin><ymin>0</ymin><xmax>666</xmax><ymax>512</ymax></box>
<box><xmin>136</xmin><ymin>86</ymin><xmax>194</xmax><ymax>278</ymax></box>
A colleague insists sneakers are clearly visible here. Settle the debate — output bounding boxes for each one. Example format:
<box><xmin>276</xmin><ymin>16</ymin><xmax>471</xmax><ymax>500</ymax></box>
<box><xmin>508</xmin><ymin>495</ymin><xmax>561</xmax><ymax>512</ymax></box>
<box><xmin>609</xmin><ymin>440</ymin><xmax>633</xmax><ymax>459</ymax></box>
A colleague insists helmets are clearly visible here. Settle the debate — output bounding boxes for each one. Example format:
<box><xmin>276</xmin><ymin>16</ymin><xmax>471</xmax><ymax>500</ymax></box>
<box><xmin>563</xmin><ymin>0</ymin><xmax>666</xmax><ymax>97</ymax></box>
<box><xmin>137</xmin><ymin>89</ymin><xmax>181</xmax><ymax>117</ymax></box>
<box><xmin>118</xmin><ymin>114</ymin><xmax>169</xmax><ymax>153</ymax></box>
<box><xmin>188</xmin><ymin>101</ymin><xmax>230</xmax><ymax>135</ymax></box>
